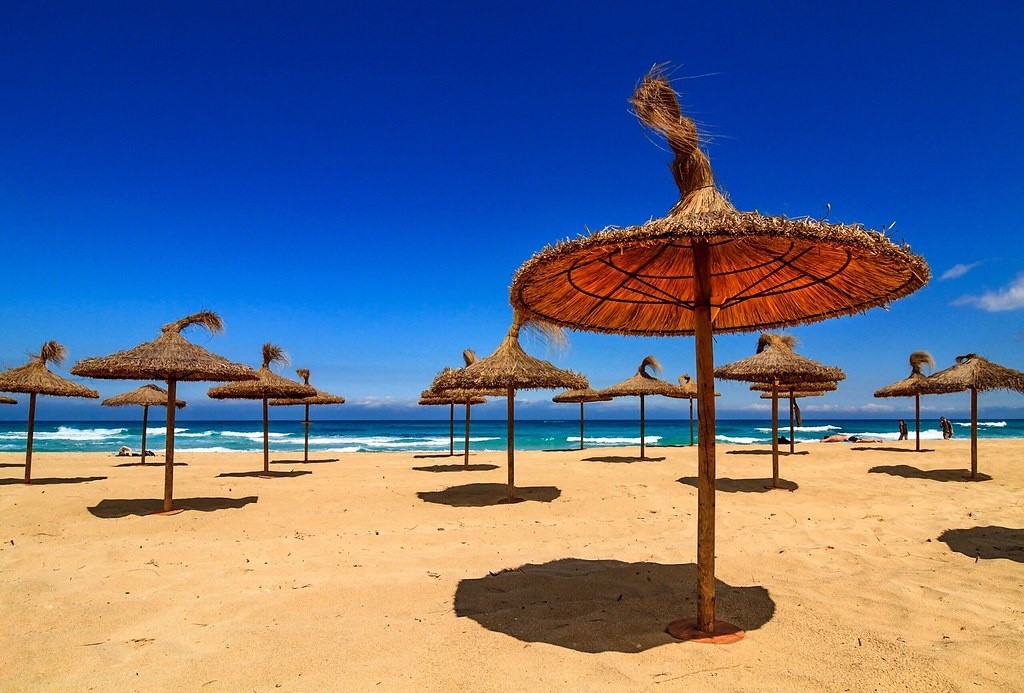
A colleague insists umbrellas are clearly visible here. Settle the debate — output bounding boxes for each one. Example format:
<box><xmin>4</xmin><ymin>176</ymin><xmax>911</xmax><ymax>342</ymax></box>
<box><xmin>510</xmin><ymin>58</ymin><xmax>929</xmax><ymax>643</ymax></box>
<box><xmin>0</xmin><ymin>308</ymin><xmax>1024</xmax><ymax>514</ymax></box>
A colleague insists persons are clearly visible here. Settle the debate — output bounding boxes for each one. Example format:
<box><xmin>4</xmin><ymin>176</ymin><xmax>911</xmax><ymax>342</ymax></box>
<box><xmin>938</xmin><ymin>416</ymin><xmax>953</xmax><ymax>440</ymax></box>
<box><xmin>118</xmin><ymin>445</ymin><xmax>155</xmax><ymax>456</ymax></box>
<box><xmin>897</xmin><ymin>419</ymin><xmax>909</xmax><ymax>440</ymax></box>
